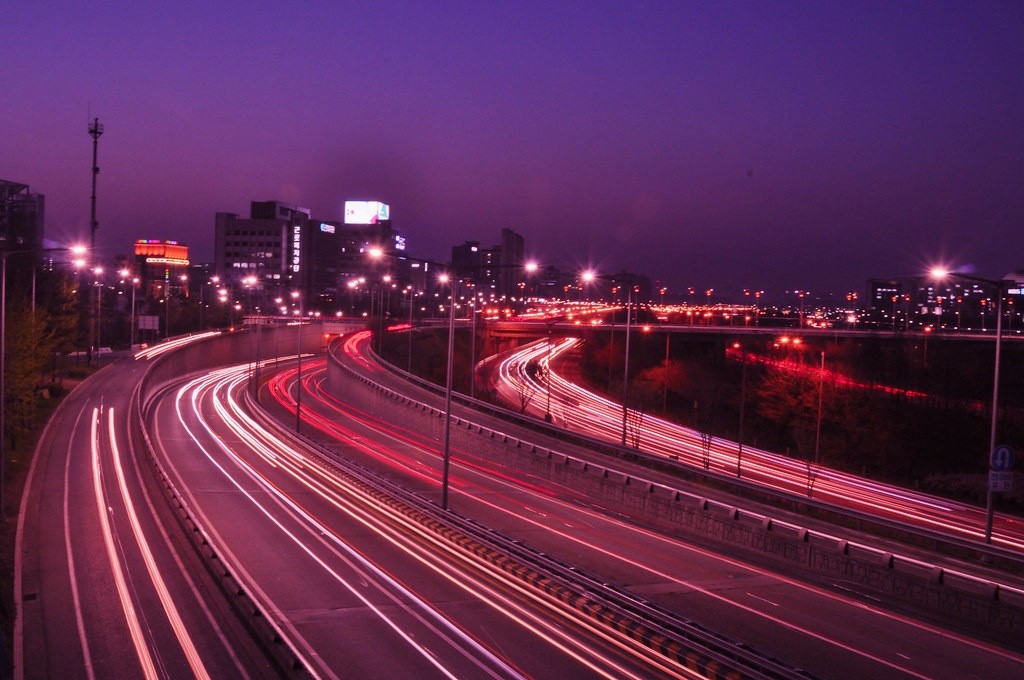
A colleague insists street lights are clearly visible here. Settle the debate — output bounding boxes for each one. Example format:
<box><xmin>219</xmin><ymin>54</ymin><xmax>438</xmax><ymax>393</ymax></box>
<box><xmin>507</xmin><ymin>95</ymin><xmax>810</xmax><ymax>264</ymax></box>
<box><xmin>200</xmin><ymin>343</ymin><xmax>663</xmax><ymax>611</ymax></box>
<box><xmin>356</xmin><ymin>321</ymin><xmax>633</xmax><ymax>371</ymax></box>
<box><xmin>632</xmin><ymin>282</ymin><xmax>1024</xmax><ymax>336</ymax></box>
<box><xmin>27</xmin><ymin>259</ymin><xmax>86</xmax><ymax>427</ymax></box>
<box><xmin>364</xmin><ymin>247</ymin><xmax>541</xmax><ymax>510</ymax></box>
<box><xmin>199</xmin><ymin>275</ymin><xmax>220</xmax><ymax>330</ymax></box>
<box><xmin>164</xmin><ymin>274</ymin><xmax>187</xmax><ymax>337</ymax></box>
<box><xmin>0</xmin><ymin>246</ymin><xmax>87</xmax><ymax>445</ymax></box>
<box><xmin>130</xmin><ymin>276</ymin><xmax>139</xmax><ymax>345</ymax></box>
<box><xmin>289</xmin><ymin>288</ymin><xmax>304</xmax><ymax>433</ymax></box>
<box><xmin>95</xmin><ymin>264</ymin><xmax>104</xmax><ymax>358</ymax></box>
<box><xmin>582</xmin><ymin>269</ymin><xmax>634</xmax><ymax>444</ymax></box>
<box><xmin>945</xmin><ymin>270</ymin><xmax>1024</xmax><ymax>564</ymax></box>
